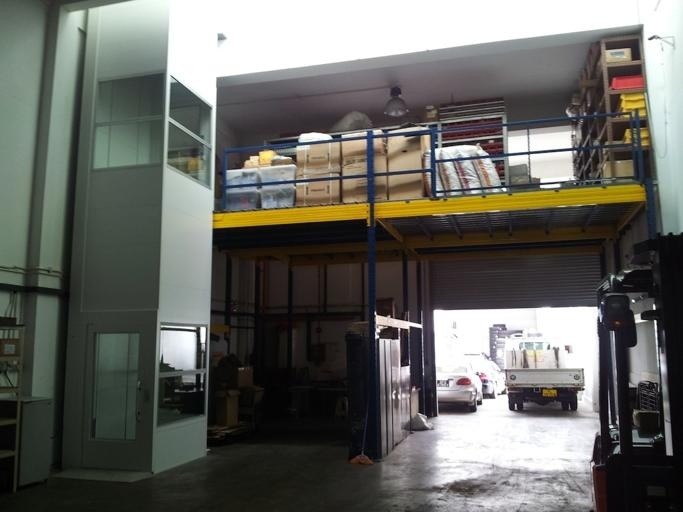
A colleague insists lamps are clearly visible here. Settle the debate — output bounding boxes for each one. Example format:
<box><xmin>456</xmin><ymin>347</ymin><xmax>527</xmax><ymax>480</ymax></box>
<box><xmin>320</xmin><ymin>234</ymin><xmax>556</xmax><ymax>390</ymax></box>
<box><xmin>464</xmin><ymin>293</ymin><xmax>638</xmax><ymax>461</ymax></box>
<box><xmin>384</xmin><ymin>86</ymin><xmax>409</xmax><ymax>117</ymax></box>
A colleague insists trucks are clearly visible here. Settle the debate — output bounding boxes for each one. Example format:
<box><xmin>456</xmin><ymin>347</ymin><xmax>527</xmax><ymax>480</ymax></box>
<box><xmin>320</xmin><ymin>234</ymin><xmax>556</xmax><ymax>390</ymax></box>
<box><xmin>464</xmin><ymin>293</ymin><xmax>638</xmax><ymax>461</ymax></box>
<box><xmin>502</xmin><ymin>331</ymin><xmax>586</xmax><ymax>413</ymax></box>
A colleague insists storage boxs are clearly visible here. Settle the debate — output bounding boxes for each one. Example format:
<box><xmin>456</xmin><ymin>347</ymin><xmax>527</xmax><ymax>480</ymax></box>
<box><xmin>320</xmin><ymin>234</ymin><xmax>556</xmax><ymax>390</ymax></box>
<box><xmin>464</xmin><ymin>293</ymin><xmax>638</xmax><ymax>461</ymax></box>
<box><xmin>225</xmin><ymin>127</ymin><xmax>430</xmax><ymax>211</ymax></box>
<box><xmin>216</xmin><ymin>366</ymin><xmax>265</xmax><ymax>427</ymax></box>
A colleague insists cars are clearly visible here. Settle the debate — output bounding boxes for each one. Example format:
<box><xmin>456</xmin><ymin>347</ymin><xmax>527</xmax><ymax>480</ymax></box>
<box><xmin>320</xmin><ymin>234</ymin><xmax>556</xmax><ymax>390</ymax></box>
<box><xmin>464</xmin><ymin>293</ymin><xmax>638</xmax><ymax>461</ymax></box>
<box><xmin>435</xmin><ymin>349</ymin><xmax>507</xmax><ymax>412</ymax></box>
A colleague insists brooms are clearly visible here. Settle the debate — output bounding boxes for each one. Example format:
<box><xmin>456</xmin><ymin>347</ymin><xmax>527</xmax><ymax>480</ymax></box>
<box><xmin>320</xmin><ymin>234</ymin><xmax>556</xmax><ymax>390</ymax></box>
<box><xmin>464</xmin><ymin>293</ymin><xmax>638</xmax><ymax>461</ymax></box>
<box><xmin>350</xmin><ymin>383</ymin><xmax>376</xmax><ymax>466</ymax></box>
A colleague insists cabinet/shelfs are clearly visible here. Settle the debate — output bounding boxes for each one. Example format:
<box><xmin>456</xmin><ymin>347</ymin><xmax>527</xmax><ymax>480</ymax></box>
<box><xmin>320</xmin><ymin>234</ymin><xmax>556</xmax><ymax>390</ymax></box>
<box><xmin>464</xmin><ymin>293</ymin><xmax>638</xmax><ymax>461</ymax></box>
<box><xmin>3</xmin><ymin>396</ymin><xmax>52</xmax><ymax>491</ymax></box>
<box><xmin>572</xmin><ymin>35</ymin><xmax>655</xmax><ymax>183</ymax></box>
<box><xmin>0</xmin><ymin>325</ymin><xmax>26</xmax><ymax>494</ymax></box>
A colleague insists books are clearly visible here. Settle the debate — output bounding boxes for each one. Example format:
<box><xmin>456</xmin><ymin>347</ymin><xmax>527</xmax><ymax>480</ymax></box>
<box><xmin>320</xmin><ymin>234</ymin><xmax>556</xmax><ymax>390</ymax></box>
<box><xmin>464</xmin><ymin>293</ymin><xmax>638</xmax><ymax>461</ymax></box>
<box><xmin>341</xmin><ymin>129</ymin><xmax>383</xmax><ymax>138</ymax></box>
